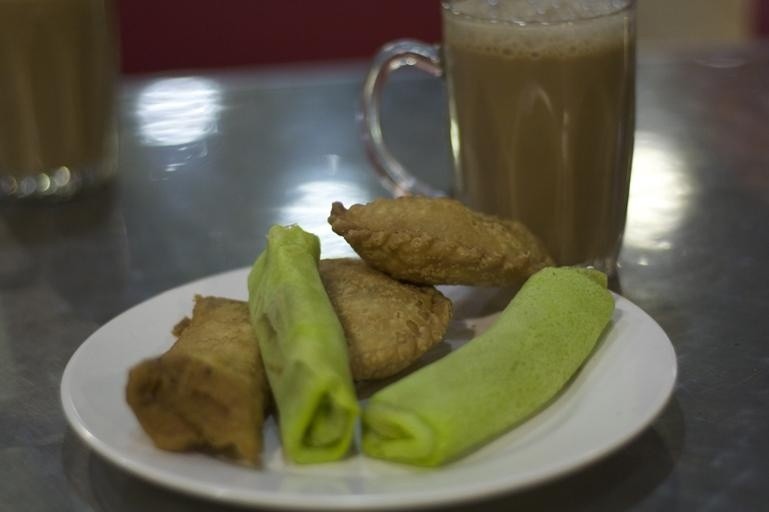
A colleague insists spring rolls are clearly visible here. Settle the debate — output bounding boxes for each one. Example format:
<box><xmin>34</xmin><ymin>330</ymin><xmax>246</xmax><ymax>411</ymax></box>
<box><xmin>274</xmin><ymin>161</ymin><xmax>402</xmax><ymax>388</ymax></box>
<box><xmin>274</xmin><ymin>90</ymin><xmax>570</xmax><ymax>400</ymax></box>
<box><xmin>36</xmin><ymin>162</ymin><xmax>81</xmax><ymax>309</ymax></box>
<box><xmin>245</xmin><ymin>224</ymin><xmax>359</xmax><ymax>460</ymax></box>
<box><xmin>358</xmin><ymin>263</ymin><xmax>616</xmax><ymax>466</ymax></box>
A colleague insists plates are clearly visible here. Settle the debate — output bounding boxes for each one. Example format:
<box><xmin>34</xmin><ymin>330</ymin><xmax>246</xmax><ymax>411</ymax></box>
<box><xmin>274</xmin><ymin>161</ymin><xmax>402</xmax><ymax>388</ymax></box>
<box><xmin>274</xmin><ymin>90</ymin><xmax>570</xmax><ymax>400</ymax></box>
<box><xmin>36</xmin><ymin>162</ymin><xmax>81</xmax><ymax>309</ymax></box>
<box><xmin>57</xmin><ymin>257</ymin><xmax>679</xmax><ymax>511</ymax></box>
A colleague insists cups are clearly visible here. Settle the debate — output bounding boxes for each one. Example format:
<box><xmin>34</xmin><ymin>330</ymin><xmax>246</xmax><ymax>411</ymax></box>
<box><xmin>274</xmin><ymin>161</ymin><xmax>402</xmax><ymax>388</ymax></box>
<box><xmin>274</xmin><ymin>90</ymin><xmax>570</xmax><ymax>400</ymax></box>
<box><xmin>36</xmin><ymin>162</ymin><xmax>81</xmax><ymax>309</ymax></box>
<box><xmin>359</xmin><ymin>0</ymin><xmax>638</xmax><ymax>279</ymax></box>
<box><xmin>0</xmin><ymin>0</ymin><xmax>120</xmax><ymax>197</ymax></box>
